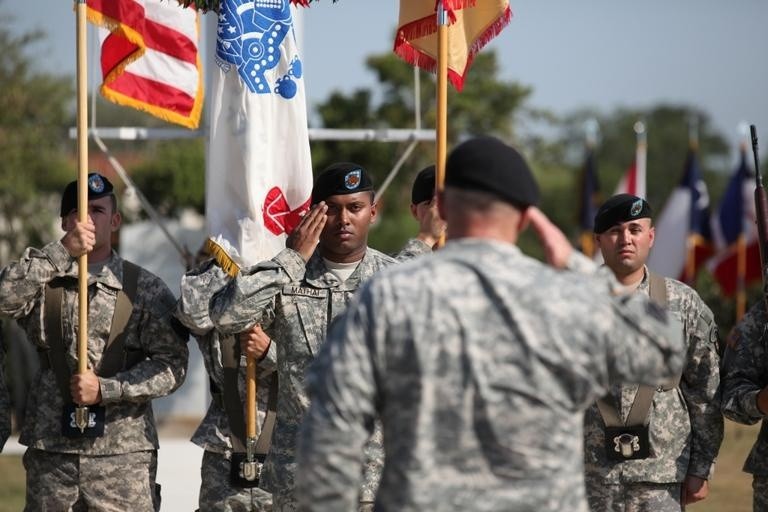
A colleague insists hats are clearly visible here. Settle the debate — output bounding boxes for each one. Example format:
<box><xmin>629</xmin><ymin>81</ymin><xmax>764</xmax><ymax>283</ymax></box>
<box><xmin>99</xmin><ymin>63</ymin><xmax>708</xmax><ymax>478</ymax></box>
<box><xmin>444</xmin><ymin>137</ymin><xmax>540</xmax><ymax>208</ymax></box>
<box><xmin>60</xmin><ymin>173</ymin><xmax>113</xmax><ymax>216</ymax></box>
<box><xmin>594</xmin><ymin>194</ymin><xmax>653</xmax><ymax>233</ymax></box>
<box><xmin>310</xmin><ymin>163</ymin><xmax>375</xmax><ymax>206</ymax></box>
<box><xmin>410</xmin><ymin>165</ymin><xmax>437</xmax><ymax>203</ymax></box>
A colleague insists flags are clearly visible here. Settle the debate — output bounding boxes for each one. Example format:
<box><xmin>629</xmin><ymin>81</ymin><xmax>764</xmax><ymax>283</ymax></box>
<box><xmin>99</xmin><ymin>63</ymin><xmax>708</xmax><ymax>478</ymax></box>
<box><xmin>578</xmin><ymin>115</ymin><xmax>761</xmax><ymax>325</ymax></box>
<box><xmin>393</xmin><ymin>1</ymin><xmax>515</xmax><ymax>91</ymax></box>
<box><xmin>206</xmin><ymin>0</ymin><xmax>314</xmax><ymax>270</ymax></box>
<box><xmin>72</xmin><ymin>0</ymin><xmax>317</xmax><ymax>133</ymax></box>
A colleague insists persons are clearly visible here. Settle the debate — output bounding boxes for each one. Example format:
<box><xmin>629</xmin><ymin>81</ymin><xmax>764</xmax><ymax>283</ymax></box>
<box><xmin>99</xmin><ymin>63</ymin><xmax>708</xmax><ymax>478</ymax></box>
<box><xmin>574</xmin><ymin>194</ymin><xmax>722</xmax><ymax>511</ymax></box>
<box><xmin>0</xmin><ymin>171</ymin><xmax>187</xmax><ymax>511</ymax></box>
<box><xmin>209</xmin><ymin>161</ymin><xmax>396</xmax><ymax>510</ymax></box>
<box><xmin>394</xmin><ymin>164</ymin><xmax>447</xmax><ymax>262</ymax></box>
<box><xmin>292</xmin><ymin>131</ymin><xmax>689</xmax><ymax>510</ymax></box>
<box><xmin>175</xmin><ymin>234</ymin><xmax>279</xmax><ymax>512</ymax></box>
<box><xmin>721</xmin><ymin>292</ymin><xmax>766</xmax><ymax>510</ymax></box>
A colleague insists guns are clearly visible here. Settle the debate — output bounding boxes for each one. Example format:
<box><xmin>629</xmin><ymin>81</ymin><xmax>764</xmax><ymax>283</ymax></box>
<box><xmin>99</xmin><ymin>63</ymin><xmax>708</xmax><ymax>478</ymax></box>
<box><xmin>751</xmin><ymin>125</ymin><xmax>768</xmax><ymax>342</ymax></box>
